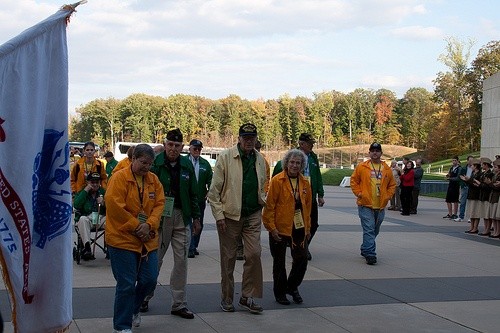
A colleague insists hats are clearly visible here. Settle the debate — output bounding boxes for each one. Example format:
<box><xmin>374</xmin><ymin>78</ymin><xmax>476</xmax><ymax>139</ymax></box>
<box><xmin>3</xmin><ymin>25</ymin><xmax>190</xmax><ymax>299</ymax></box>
<box><xmin>239</xmin><ymin>124</ymin><xmax>257</xmax><ymax>136</ymax></box>
<box><xmin>369</xmin><ymin>143</ymin><xmax>381</xmax><ymax>150</ymax></box>
<box><xmin>469</xmin><ymin>155</ymin><xmax>500</xmax><ymax>166</ymax></box>
<box><xmin>190</xmin><ymin>140</ymin><xmax>203</xmax><ymax>148</ymax></box>
<box><xmin>104</xmin><ymin>152</ymin><xmax>113</xmax><ymax>157</ymax></box>
<box><xmin>87</xmin><ymin>172</ymin><xmax>101</xmax><ymax>181</ymax></box>
<box><xmin>167</xmin><ymin>128</ymin><xmax>183</xmax><ymax>142</ymax></box>
<box><xmin>299</xmin><ymin>133</ymin><xmax>315</xmax><ymax>140</ymax></box>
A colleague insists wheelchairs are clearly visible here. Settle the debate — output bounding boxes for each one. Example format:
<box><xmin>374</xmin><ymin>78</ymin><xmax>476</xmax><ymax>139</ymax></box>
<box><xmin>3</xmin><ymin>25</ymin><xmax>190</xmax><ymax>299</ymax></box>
<box><xmin>72</xmin><ymin>210</ymin><xmax>110</xmax><ymax>264</ymax></box>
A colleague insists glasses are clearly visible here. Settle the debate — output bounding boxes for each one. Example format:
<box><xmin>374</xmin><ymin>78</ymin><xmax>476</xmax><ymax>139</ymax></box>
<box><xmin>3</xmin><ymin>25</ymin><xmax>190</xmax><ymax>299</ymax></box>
<box><xmin>194</xmin><ymin>148</ymin><xmax>201</xmax><ymax>151</ymax></box>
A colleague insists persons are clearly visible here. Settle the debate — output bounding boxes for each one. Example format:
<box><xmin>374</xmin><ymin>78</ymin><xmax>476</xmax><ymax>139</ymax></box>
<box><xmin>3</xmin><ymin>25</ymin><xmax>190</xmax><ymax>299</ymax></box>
<box><xmin>262</xmin><ymin>148</ymin><xmax>312</xmax><ymax>305</ymax></box>
<box><xmin>140</xmin><ymin>128</ymin><xmax>202</xmax><ymax>319</ymax></box>
<box><xmin>104</xmin><ymin>144</ymin><xmax>165</xmax><ymax>333</ymax></box>
<box><xmin>387</xmin><ymin>156</ymin><xmax>423</xmax><ymax>216</ymax></box>
<box><xmin>272</xmin><ymin>133</ymin><xmax>325</xmax><ymax>259</ymax></box>
<box><xmin>70</xmin><ymin>142</ymin><xmax>164</xmax><ymax>261</ymax></box>
<box><xmin>236</xmin><ymin>141</ymin><xmax>270</xmax><ymax>259</ymax></box>
<box><xmin>443</xmin><ymin>155</ymin><xmax>500</xmax><ymax>238</ymax></box>
<box><xmin>208</xmin><ymin>122</ymin><xmax>266</xmax><ymax>312</ymax></box>
<box><xmin>184</xmin><ymin>139</ymin><xmax>213</xmax><ymax>258</ymax></box>
<box><xmin>350</xmin><ymin>141</ymin><xmax>395</xmax><ymax>263</ymax></box>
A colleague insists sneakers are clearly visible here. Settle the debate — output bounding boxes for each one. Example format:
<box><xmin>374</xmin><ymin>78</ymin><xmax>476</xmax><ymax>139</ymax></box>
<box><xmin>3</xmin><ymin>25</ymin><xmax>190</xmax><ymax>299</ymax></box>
<box><xmin>287</xmin><ymin>287</ymin><xmax>303</xmax><ymax>303</ymax></box>
<box><xmin>238</xmin><ymin>296</ymin><xmax>263</xmax><ymax>312</ymax></box>
<box><xmin>220</xmin><ymin>301</ymin><xmax>234</xmax><ymax>311</ymax></box>
<box><xmin>276</xmin><ymin>294</ymin><xmax>290</xmax><ymax>305</ymax></box>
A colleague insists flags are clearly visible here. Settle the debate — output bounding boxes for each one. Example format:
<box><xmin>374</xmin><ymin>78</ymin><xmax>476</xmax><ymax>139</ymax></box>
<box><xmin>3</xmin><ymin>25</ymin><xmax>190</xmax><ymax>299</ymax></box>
<box><xmin>0</xmin><ymin>8</ymin><xmax>73</xmax><ymax>333</ymax></box>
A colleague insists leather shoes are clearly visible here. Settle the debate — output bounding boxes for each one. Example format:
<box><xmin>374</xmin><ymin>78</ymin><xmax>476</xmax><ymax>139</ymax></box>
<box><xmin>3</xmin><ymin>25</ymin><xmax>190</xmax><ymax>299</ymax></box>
<box><xmin>141</xmin><ymin>301</ymin><xmax>149</xmax><ymax>312</ymax></box>
<box><xmin>171</xmin><ymin>308</ymin><xmax>194</xmax><ymax>319</ymax></box>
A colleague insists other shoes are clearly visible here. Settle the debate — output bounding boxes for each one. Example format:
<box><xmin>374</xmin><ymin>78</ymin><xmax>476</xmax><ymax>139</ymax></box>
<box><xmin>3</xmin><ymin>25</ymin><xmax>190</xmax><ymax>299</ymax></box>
<box><xmin>85</xmin><ymin>251</ymin><xmax>91</xmax><ymax>257</ymax></box>
<box><xmin>361</xmin><ymin>251</ymin><xmax>376</xmax><ymax>264</ymax></box>
<box><xmin>236</xmin><ymin>247</ymin><xmax>243</xmax><ymax>260</ymax></box>
<box><xmin>308</xmin><ymin>251</ymin><xmax>311</xmax><ymax>260</ymax></box>
<box><xmin>389</xmin><ymin>205</ymin><xmax>417</xmax><ymax>215</ymax></box>
<box><xmin>131</xmin><ymin>316</ymin><xmax>139</xmax><ymax>327</ymax></box>
<box><xmin>188</xmin><ymin>249</ymin><xmax>199</xmax><ymax>258</ymax></box>
<box><xmin>443</xmin><ymin>213</ymin><xmax>500</xmax><ymax>238</ymax></box>
<box><xmin>112</xmin><ymin>328</ymin><xmax>133</xmax><ymax>333</ymax></box>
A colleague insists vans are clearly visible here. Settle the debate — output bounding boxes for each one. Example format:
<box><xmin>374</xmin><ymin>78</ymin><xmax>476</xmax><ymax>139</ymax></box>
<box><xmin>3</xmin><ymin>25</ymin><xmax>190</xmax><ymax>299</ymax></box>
<box><xmin>114</xmin><ymin>141</ymin><xmax>164</xmax><ymax>163</ymax></box>
